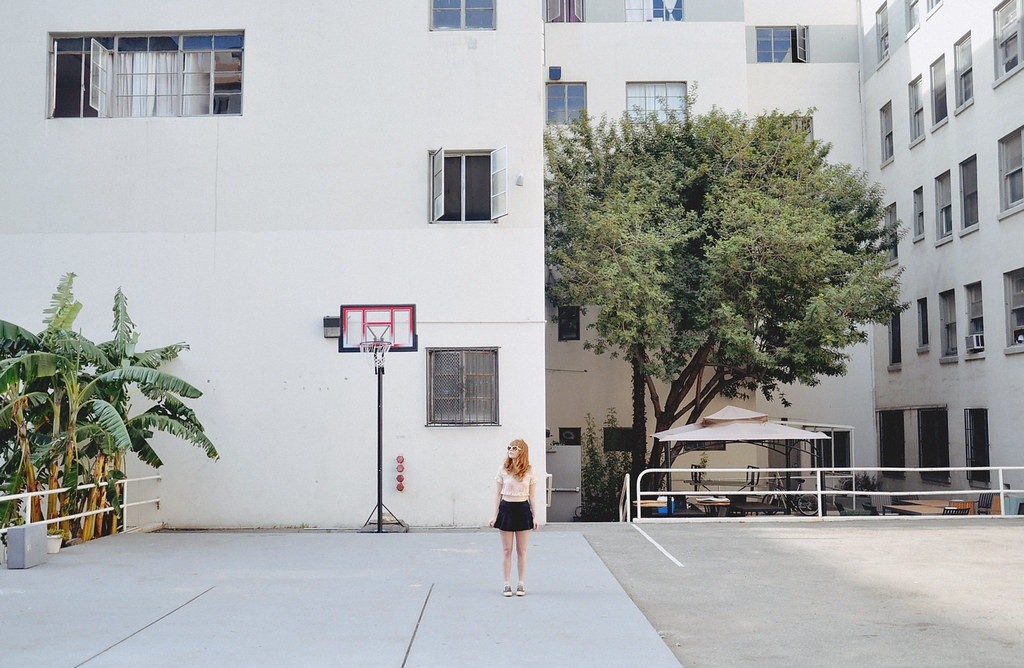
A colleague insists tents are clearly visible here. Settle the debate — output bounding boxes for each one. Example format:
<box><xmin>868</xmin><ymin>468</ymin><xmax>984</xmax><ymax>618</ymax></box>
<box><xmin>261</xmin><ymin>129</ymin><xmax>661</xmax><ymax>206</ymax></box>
<box><xmin>650</xmin><ymin>406</ymin><xmax>831</xmax><ymax>518</ymax></box>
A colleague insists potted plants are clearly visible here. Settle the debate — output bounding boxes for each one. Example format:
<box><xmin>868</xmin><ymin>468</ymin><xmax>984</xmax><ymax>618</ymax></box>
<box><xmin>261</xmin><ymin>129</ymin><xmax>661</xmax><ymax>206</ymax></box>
<box><xmin>47</xmin><ymin>529</ymin><xmax>69</xmax><ymax>553</ymax></box>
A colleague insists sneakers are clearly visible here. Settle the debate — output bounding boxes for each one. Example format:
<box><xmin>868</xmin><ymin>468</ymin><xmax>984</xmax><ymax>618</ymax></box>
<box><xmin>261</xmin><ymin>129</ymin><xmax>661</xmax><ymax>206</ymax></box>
<box><xmin>503</xmin><ymin>584</ymin><xmax>512</xmax><ymax>596</ymax></box>
<box><xmin>516</xmin><ymin>585</ymin><xmax>525</xmax><ymax>595</ymax></box>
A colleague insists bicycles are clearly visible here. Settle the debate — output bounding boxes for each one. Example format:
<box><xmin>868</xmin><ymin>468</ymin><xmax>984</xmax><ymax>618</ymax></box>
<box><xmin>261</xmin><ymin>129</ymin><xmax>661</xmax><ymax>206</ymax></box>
<box><xmin>761</xmin><ymin>478</ymin><xmax>819</xmax><ymax>516</ymax></box>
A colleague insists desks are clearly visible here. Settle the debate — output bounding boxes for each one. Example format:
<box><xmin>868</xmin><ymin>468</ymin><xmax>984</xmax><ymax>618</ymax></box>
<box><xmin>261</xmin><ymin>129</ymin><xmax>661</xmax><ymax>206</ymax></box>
<box><xmin>671</xmin><ymin>509</ymin><xmax>706</xmax><ymax>516</ymax></box>
<box><xmin>633</xmin><ymin>499</ymin><xmax>668</xmax><ymax>516</ymax></box>
<box><xmin>901</xmin><ymin>500</ymin><xmax>950</xmax><ymax>508</ymax></box>
<box><xmin>692</xmin><ymin>503</ymin><xmax>730</xmax><ymax>517</ymax></box>
<box><xmin>731</xmin><ymin>502</ymin><xmax>786</xmax><ymax>515</ymax></box>
<box><xmin>882</xmin><ymin>504</ymin><xmax>957</xmax><ymax>515</ymax></box>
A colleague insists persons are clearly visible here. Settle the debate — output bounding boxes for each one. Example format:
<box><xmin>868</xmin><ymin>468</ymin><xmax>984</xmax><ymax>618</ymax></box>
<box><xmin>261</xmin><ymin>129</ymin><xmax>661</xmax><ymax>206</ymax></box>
<box><xmin>488</xmin><ymin>439</ymin><xmax>539</xmax><ymax>596</ymax></box>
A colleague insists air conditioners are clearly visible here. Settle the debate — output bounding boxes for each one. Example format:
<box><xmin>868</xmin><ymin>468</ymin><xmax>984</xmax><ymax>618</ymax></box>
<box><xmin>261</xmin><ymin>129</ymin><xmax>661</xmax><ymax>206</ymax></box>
<box><xmin>965</xmin><ymin>334</ymin><xmax>985</xmax><ymax>351</ymax></box>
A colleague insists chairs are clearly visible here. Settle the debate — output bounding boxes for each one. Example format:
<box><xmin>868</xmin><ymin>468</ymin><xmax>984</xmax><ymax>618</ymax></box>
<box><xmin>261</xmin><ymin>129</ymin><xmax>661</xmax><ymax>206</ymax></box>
<box><xmin>943</xmin><ymin>493</ymin><xmax>1002</xmax><ymax>515</ymax></box>
<box><xmin>834</xmin><ymin>495</ymin><xmax>918</xmax><ymax>516</ymax></box>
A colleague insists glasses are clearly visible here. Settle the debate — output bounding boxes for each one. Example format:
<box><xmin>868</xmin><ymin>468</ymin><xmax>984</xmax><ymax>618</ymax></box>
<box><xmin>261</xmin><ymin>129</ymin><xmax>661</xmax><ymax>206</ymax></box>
<box><xmin>508</xmin><ymin>445</ymin><xmax>522</xmax><ymax>451</ymax></box>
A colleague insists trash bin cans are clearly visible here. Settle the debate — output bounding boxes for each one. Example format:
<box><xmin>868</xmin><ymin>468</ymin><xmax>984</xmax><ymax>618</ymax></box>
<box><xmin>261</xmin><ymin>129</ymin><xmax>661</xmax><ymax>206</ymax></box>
<box><xmin>6</xmin><ymin>522</ymin><xmax>48</xmax><ymax>569</ymax></box>
<box><xmin>657</xmin><ymin>496</ymin><xmax>675</xmax><ymax>513</ymax></box>
